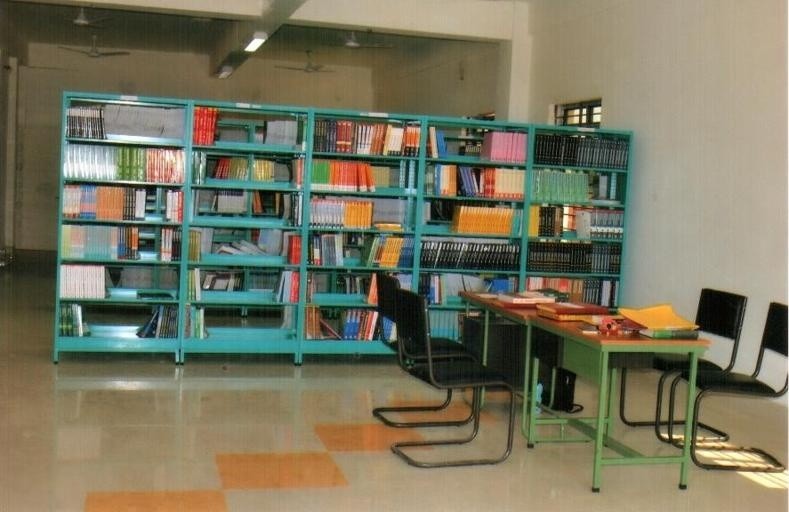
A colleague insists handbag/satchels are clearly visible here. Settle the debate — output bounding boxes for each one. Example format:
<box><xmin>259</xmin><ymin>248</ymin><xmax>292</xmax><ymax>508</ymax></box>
<box><xmin>540</xmin><ymin>363</ymin><xmax>576</xmax><ymax>411</ymax></box>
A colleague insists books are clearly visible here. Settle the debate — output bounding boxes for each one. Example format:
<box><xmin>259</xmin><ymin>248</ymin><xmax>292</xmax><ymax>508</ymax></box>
<box><xmin>474</xmin><ymin>287</ymin><xmax>625</xmax><ymax>327</ymax></box>
<box><xmin>418</xmin><ymin>127</ymin><xmax>527</xmax><ymax>344</ymax></box>
<box><xmin>186</xmin><ymin>107</ymin><xmax>309</xmax><ymax>340</ymax></box>
<box><xmin>57</xmin><ymin>103</ymin><xmax>187</xmax><ymax>337</ymax></box>
<box><xmin>305</xmin><ymin>117</ymin><xmax>421</xmax><ymax>342</ymax></box>
<box><xmin>618</xmin><ymin>302</ymin><xmax>701</xmax><ymax>341</ymax></box>
<box><xmin>578</xmin><ymin>326</ymin><xmax>598</xmax><ymax>334</ymax></box>
<box><xmin>526</xmin><ymin>134</ymin><xmax>629</xmax><ymax>307</ymax></box>
